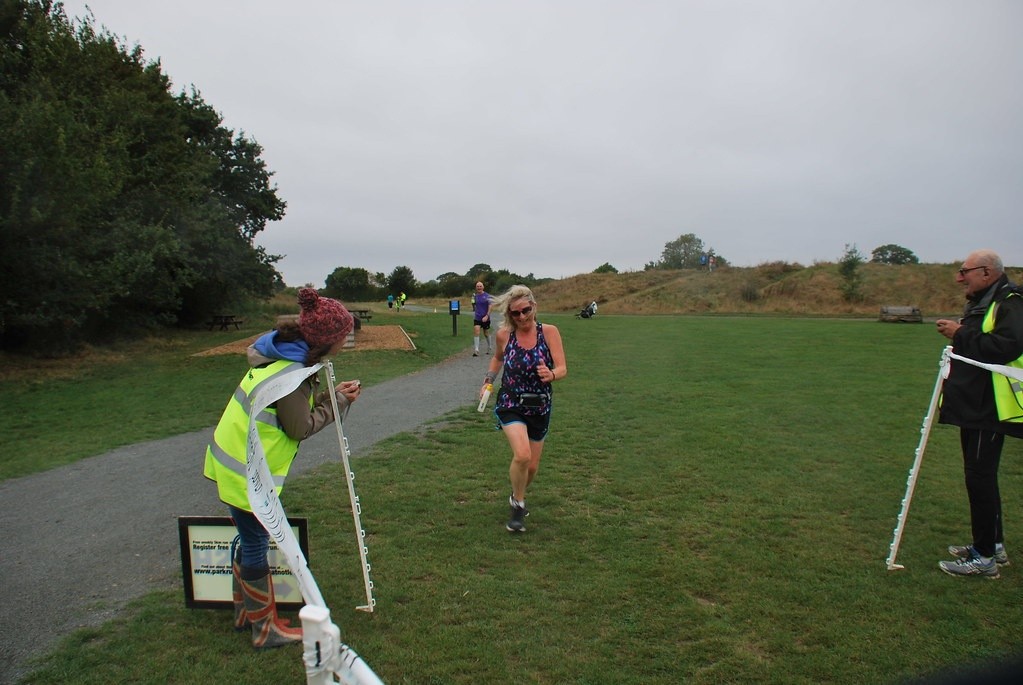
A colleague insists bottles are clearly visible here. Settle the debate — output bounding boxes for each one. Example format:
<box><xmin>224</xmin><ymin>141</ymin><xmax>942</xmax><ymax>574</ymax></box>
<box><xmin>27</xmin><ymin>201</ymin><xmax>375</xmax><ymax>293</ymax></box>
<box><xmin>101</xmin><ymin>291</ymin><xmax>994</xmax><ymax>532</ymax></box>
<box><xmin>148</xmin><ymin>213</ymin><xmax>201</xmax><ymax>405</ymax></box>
<box><xmin>477</xmin><ymin>383</ymin><xmax>493</xmax><ymax>412</ymax></box>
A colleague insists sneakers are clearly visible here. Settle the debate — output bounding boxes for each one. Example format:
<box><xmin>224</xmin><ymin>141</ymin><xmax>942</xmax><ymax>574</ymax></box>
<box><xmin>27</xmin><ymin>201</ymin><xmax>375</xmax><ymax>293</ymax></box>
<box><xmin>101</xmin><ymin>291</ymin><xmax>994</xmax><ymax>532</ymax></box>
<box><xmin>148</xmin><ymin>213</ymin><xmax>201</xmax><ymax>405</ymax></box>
<box><xmin>506</xmin><ymin>503</ymin><xmax>531</xmax><ymax>534</ymax></box>
<box><xmin>939</xmin><ymin>547</ymin><xmax>1000</xmax><ymax>581</ymax></box>
<box><xmin>509</xmin><ymin>492</ymin><xmax>530</xmax><ymax>517</ymax></box>
<box><xmin>486</xmin><ymin>347</ymin><xmax>491</xmax><ymax>354</ymax></box>
<box><xmin>948</xmin><ymin>541</ymin><xmax>1010</xmax><ymax>568</ymax></box>
<box><xmin>472</xmin><ymin>350</ymin><xmax>480</xmax><ymax>356</ymax></box>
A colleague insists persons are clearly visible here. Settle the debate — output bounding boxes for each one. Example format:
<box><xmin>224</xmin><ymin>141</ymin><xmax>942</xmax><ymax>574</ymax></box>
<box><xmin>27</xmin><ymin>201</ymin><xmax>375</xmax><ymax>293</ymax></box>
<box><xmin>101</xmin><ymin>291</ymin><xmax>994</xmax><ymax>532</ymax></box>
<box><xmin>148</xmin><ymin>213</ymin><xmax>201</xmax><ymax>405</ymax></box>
<box><xmin>204</xmin><ymin>289</ymin><xmax>361</xmax><ymax>648</ymax></box>
<box><xmin>470</xmin><ymin>293</ymin><xmax>476</xmax><ymax>311</ymax></box>
<box><xmin>472</xmin><ymin>281</ymin><xmax>492</xmax><ymax>356</ymax></box>
<box><xmin>387</xmin><ymin>293</ymin><xmax>394</xmax><ymax>308</ymax></box>
<box><xmin>935</xmin><ymin>251</ymin><xmax>1023</xmax><ymax>580</ymax></box>
<box><xmin>400</xmin><ymin>291</ymin><xmax>406</xmax><ymax>308</ymax></box>
<box><xmin>480</xmin><ymin>284</ymin><xmax>568</xmax><ymax>532</ymax></box>
<box><xmin>700</xmin><ymin>254</ymin><xmax>715</xmax><ymax>272</ymax></box>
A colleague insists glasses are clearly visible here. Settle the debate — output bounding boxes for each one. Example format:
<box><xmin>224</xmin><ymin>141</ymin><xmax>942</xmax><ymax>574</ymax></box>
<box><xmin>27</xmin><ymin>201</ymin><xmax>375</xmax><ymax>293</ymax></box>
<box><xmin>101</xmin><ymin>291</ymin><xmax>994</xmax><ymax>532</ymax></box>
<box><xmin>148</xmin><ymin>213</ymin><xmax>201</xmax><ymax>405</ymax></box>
<box><xmin>960</xmin><ymin>266</ymin><xmax>990</xmax><ymax>277</ymax></box>
<box><xmin>508</xmin><ymin>304</ymin><xmax>532</xmax><ymax>317</ymax></box>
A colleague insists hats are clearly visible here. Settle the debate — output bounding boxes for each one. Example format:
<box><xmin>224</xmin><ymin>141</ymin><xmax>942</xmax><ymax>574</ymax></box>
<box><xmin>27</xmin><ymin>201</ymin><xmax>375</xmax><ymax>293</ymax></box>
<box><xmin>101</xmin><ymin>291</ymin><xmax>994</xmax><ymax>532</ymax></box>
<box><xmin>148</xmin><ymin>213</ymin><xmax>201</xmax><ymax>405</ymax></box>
<box><xmin>298</xmin><ymin>288</ymin><xmax>355</xmax><ymax>345</ymax></box>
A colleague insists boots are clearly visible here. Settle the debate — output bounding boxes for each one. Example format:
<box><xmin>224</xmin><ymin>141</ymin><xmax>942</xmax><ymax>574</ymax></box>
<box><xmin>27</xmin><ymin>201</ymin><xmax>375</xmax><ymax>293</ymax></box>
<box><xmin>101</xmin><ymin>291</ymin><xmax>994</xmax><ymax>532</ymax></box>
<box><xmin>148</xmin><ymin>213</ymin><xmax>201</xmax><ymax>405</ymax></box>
<box><xmin>232</xmin><ymin>560</ymin><xmax>289</xmax><ymax>632</ymax></box>
<box><xmin>241</xmin><ymin>570</ymin><xmax>301</xmax><ymax>648</ymax></box>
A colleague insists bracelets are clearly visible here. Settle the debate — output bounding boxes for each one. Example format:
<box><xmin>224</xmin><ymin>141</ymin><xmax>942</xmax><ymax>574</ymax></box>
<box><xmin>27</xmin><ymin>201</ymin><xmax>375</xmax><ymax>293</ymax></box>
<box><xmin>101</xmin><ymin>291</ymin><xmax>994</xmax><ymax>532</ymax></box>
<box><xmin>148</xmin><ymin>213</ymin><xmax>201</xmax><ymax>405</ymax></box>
<box><xmin>487</xmin><ymin>314</ymin><xmax>489</xmax><ymax>316</ymax></box>
<box><xmin>550</xmin><ymin>370</ymin><xmax>555</xmax><ymax>380</ymax></box>
